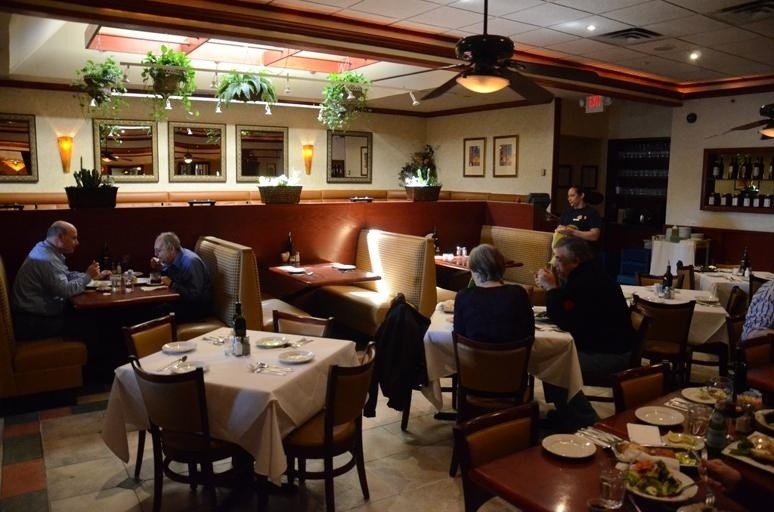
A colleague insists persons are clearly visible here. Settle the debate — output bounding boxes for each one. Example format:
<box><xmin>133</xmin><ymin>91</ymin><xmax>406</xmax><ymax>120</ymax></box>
<box><xmin>555</xmin><ymin>184</ymin><xmax>602</xmax><ymax>244</ymax></box>
<box><xmin>8</xmin><ymin>219</ymin><xmax>119</xmax><ymax>393</ymax></box>
<box><xmin>532</xmin><ymin>232</ymin><xmax>635</xmax><ymax>423</ymax></box>
<box><xmin>738</xmin><ymin>277</ymin><xmax>773</xmax><ymax>340</ymax></box>
<box><xmin>146</xmin><ymin>229</ymin><xmax>214</xmax><ymax>326</ymax></box>
<box><xmin>451</xmin><ymin>246</ymin><xmax>533</xmax><ymax>399</ymax></box>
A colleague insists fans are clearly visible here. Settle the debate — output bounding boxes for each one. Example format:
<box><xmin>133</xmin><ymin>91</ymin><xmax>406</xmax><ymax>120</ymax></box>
<box><xmin>363</xmin><ymin>0</ymin><xmax>606</xmax><ymax>106</ymax></box>
<box><xmin>101</xmin><ymin>127</ymin><xmax>132</xmax><ymax>162</ymax></box>
<box><xmin>700</xmin><ymin>97</ymin><xmax>773</xmax><ymax>143</ymax></box>
<box><xmin>176</xmin><ymin>148</ymin><xmax>207</xmax><ymax>160</ymax></box>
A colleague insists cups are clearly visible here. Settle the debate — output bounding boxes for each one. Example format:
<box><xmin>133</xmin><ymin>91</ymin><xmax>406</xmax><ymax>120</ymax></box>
<box><xmin>708</xmin><ymin>285</ymin><xmax>717</xmax><ymax>299</ymax></box>
<box><xmin>610</xmin><ymin>150</ymin><xmax>669</xmax><ymax>222</ymax></box>
<box><xmin>586</xmin><ymin>498</ymin><xmax>609</xmax><ymax>512</ymax></box>
<box><xmin>598</xmin><ymin>459</ymin><xmax>629</xmax><ymax>509</ymax></box>
<box><xmin>685</xmin><ymin>404</ymin><xmax>713</xmax><ymax>434</ymax></box>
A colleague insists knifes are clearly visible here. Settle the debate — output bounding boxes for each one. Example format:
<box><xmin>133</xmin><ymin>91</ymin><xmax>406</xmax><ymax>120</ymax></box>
<box><xmin>578</xmin><ymin>426</ymin><xmax>625</xmax><ymax>447</ymax></box>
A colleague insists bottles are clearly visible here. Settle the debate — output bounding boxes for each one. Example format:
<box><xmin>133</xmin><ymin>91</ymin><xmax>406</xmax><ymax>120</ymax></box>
<box><xmin>456</xmin><ymin>246</ymin><xmax>468</xmax><ymax>267</ymax></box>
<box><xmin>287</xmin><ymin>232</ymin><xmax>300</xmax><ymax>262</ymax></box>
<box><xmin>654</xmin><ymin>283</ymin><xmax>672</xmax><ymax>298</ymax></box>
<box><xmin>661</xmin><ymin>266</ymin><xmax>672</xmax><ymax>291</ymax></box>
<box><xmin>704</xmin><ymin>374</ymin><xmax>761</xmax><ymax>458</ymax></box>
<box><xmin>432</xmin><ymin>226</ymin><xmax>441</xmax><ymax>255</ymax></box>
<box><xmin>707</xmin><ymin>153</ymin><xmax>773</xmax><ymax>208</ymax></box>
<box><xmin>102</xmin><ymin>256</ymin><xmax>125</xmax><ymax>274</ymax></box>
<box><xmin>231</xmin><ymin>302</ymin><xmax>245</xmax><ymax>340</ymax></box>
<box><xmin>737</xmin><ymin>246</ymin><xmax>753</xmax><ymax>278</ymax></box>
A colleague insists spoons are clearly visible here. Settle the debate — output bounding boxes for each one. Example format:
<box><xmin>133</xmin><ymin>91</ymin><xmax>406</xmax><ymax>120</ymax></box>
<box><xmin>671</xmin><ymin>477</ymin><xmax>703</xmax><ymax>497</ymax></box>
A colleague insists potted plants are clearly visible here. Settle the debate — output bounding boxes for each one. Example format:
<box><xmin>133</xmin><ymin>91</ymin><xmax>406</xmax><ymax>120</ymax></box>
<box><xmin>315</xmin><ymin>70</ymin><xmax>374</xmax><ymax>137</ymax></box>
<box><xmin>138</xmin><ymin>44</ymin><xmax>201</xmax><ymax>130</ymax></box>
<box><xmin>63</xmin><ymin>154</ymin><xmax>119</xmax><ymax>207</ymax></box>
<box><xmin>211</xmin><ymin>68</ymin><xmax>276</xmax><ymax>121</ymax></box>
<box><xmin>72</xmin><ymin>50</ymin><xmax>129</xmax><ymax>143</ymax></box>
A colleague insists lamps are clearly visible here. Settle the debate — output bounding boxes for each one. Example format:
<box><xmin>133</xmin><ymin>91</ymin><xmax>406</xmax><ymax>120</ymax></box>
<box><xmin>577</xmin><ymin>89</ymin><xmax>613</xmax><ymax>114</ymax></box>
<box><xmin>758</xmin><ymin>126</ymin><xmax>773</xmax><ymax>139</ymax></box>
<box><xmin>183</xmin><ymin>158</ymin><xmax>193</xmax><ymax>163</ymax></box>
<box><xmin>102</xmin><ymin>157</ymin><xmax>114</xmax><ymax>163</ymax></box>
<box><xmin>4</xmin><ymin>158</ymin><xmax>27</xmax><ymax>174</ymax></box>
<box><xmin>81</xmin><ymin>22</ymin><xmax>385</xmax><ymax>78</ymax></box>
<box><xmin>453</xmin><ymin>63</ymin><xmax>514</xmax><ymax>96</ymax></box>
<box><xmin>302</xmin><ymin>141</ymin><xmax>316</xmax><ymax>178</ymax></box>
<box><xmin>57</xmin><ymin>133</ymin><xmax>75</xmax><ymax>174</ymax></box>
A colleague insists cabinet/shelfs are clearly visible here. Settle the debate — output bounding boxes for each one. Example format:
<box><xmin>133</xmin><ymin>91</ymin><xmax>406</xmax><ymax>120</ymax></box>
<box><xmin>696</xmin><ymin>142</ymin><xmax>773</xmax><ymax>215</ymax></box>
<box><xmin>602</xmin><ymin>132</ymin><xmax>671</xmax><ymax>228</ymax></box>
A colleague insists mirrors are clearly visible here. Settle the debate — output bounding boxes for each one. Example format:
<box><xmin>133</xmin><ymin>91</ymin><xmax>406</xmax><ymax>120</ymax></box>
<box><xmin>235</xmin><ymin>122</ymin><xmax>290</xmax><ymax>184</ymax></box>
<box><xmin>326</xmin><ymin>128</ymin><xmax>374</xmax><ymax>185</ymax></box>
<box><xmin>167</xmin><ymin>121</ymin><xmax>228</xmax><ymax>184</ymax></box>
<box><xmin>91</xmin><ymin>116</ymin><xmax>160</xmax><ymax>185</ymax></box>
<box><xmin>1</xmin><ymin>110</ymin><xmax>41</xmax><ymax>184</ymax></box>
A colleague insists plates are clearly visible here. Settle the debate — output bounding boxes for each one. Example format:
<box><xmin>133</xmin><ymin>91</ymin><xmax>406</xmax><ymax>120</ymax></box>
<box><xmin>286</xmin><ymin>268</ymin><xmax>304</xmax><ymax>273</ymax></box>
<box><xmin>541</xmin><ymin>433</ymin><xmax>596</xmax><ymax>458</ymax></box>
<box><xmin>620</xmin><ymin>285</ymin><xmax>649</xmax><ymax>299</ymax></box>
<box><xmin>623</xmin><ymin>464</ymin><xmax>698</xmax><ymax>502</ymax></box>
<box><xmin>676</xmin><ymin>505</ymin><xmax>717</xmax><ymax>512</ymax></box>
<box><xmin>755</xmin><ymin>409</ymin><xmax>773</xmax><ymax>430</ymax></box>
<box><xmin>696</xmin><ymin>295</ymin><xmax>720</xmax><ymax>303</ymax></box>
<box><xmin>334</xmin><ymin>264</ymin><xmax>356</xmax><ymax>270</ymax></box>
<box><xmin>707</xmin><ymin>271</ymin><xmax>723</xmax><ymax>277</ymax></box>
<box><xmin>666</xmin><ymin>225</ymin><xmax>705</xmax><ymax>242</ymax></box>
<box><xmin>161</xmin><ymin>333</ymin><xmax>314</xmax><ymax>375</ymax></box>
<box><xmin>634</xmin><ymin>406</ymin><xmax>685</xmax><ymax>426</ymax></box>
<box><xmin>721</xmin><ymin>431</ymin><xmax>774</xmax><ymax>474</ymax></box>
<box><xmin>85</xmin><ymin>268</ymin><xmax>163</xmax><ymax>293</ymax></box>
<box><xmin>681</xmin><ymin>386</ymin><xmax>717</xmax><ymax>404</ymax></box>
<box><xmin>659</xmin><ymin>433</ymin><xmax>705</xmax><ymax>451</ymax></box>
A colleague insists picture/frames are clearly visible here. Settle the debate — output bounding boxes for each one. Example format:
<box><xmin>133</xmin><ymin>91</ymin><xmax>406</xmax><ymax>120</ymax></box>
<box><xmin>360</xmin><ymin>145</ymin><xmax>368</xmax><ymax>177</ymax></box>
<box><xmin>557</xmin><ymin>163</ymin><xmax>572</xmax><ymax>189</ymax></box>
<box><xmin>579</xmin><ymin>163</ymin><xmax>599</xmax><ymax>191</ymax></box>
<box><xmin>491</xmin><ymin>132</ymin><xmax>520</xmax><ymax>179</ymax></box>
<box><xmin>462</xmin><ymin>136</ymin><xmax>488</xmax><ymax>179</ymax></box>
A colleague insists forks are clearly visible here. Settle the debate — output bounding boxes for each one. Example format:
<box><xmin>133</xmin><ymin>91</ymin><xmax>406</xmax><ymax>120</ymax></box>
<box><xmin>702</xmin><ymin>482</ymin><xmax>715</xmax><ymax>505</ymax></box>
